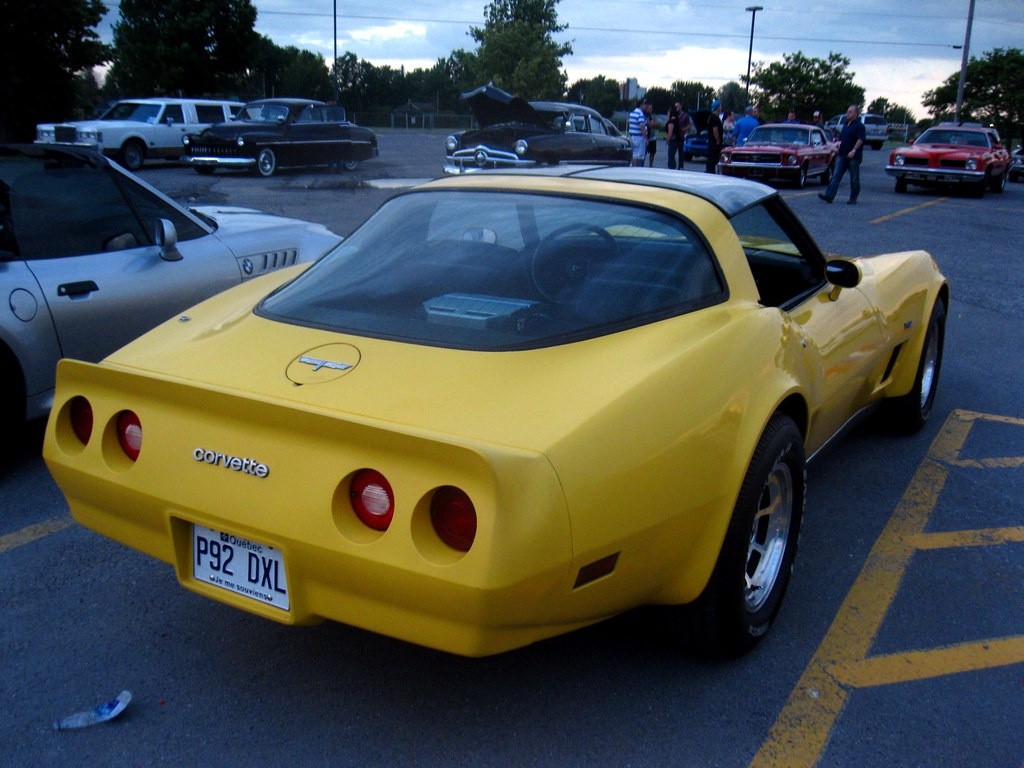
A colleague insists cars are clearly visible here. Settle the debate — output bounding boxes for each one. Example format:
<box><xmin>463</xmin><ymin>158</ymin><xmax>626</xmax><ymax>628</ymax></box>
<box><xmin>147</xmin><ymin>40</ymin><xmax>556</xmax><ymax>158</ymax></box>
<box><xmin>718</xmin><ymin>123</ymin><xmax>839</xmax><ymax>190</ymax></box>
<box><xmin>0</xmin><ymin>141</ymin><xmax>344</xmax><ymax>478</ymax></box>
<box><xmin>44</xmin><ymin>166</ymin><xmax>949</xmax><ymax>655</ymax></box>
<box><xmin>1009</xmin><ymin>145</ymin><xmax>1023</xmax><ymax>182</ymax></box>
<box><xmin>179</xmin><ymin>98</ymin><xmax>379</xmax><ymax>177</ymax></box>
<box><xmin>683</xmin><ymin>110</ymin><xmax>713</xmax><ymax>162</ymax></box>
<box><xmin>34</xmin><ymin>98</ymin><xmax>264</xmax><ymax>170</ymax></box>
<box><xmin>441</xmin><ymin>82</ymin><xmax>634</xmax><ymax>176</ymax></box>
<box><xmin>885</xmin><ymin>122</ymin><xmax>1010</xmax><ymax>198</ymax></box>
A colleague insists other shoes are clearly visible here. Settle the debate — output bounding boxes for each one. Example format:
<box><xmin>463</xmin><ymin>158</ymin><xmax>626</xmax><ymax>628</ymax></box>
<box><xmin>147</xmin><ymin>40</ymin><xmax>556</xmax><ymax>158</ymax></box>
<box><xmin>818</xmin><ymin>193</ymin><xmax>833</xmax><ymax>203</ymax></box>
<box><xmin>847</xmin><ymin>199</ymin><xmax>856</xmax><ymax>204</ymax></box>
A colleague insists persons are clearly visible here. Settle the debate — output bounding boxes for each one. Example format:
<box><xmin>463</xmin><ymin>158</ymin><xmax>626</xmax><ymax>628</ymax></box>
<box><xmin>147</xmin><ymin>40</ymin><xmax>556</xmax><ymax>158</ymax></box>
<box><xmin>722</xmin><ymin>107</ymin><xmax>770</xmax><ymax>148</ymax></box>
<box><xmin>627</xmin><ymin>99</ymin><xmax>660</xmax><ymax>168</ymax></box>
<box><xmin>664</xmin><ymin>103</ymin><xmax>693</xmax><ymax>171</ymax></box>
<box><xmin>817</xmin><ymin>105</ymin><xmax>866</xmax><ymax>205</ymax></box>
<box><xmin>706</xmin><ymin>100</ymin><xmax>724</xmax><ymax>174</ymax></box>
<box><xmin>811</xmin><ymin>111</ymin><xmax>825</xmax><ymax>131</ymax></box>
<box><xmin>782</xmin><ymin>112</ymin><xmax>800</xmax><ymax>142</ymax></box>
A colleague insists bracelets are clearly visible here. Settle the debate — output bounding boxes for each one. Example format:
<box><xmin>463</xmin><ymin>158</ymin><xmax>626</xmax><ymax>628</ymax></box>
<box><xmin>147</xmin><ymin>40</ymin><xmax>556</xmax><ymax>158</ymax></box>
<box><xmin>852</xmin><ymin>149</ymin><xmax>855</xmax><ymax>152</ymax></box>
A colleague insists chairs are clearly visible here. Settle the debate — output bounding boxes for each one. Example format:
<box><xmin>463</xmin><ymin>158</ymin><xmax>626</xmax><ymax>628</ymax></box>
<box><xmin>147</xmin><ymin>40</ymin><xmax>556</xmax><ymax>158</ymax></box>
<box><xmin>422</xmin><ymin>237</ymin><xmax>524</xmax><ymax>299</ymax></box>
<box><xmin>580</xmin><ymin>263</ymin><xmax>690</xmax><ymax>320</ymax></box>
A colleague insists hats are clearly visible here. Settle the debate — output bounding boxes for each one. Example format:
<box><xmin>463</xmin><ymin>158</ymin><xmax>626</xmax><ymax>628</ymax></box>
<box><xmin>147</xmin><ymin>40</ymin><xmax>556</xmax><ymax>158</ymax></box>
<box><xmin>712</xmin><ymin>99</ymin><xmax>720</xmax><ymax>111</ymax></box>
<box><xmin>746</xmin><ymin>106</ymin><xmax>753</xmax><ymax>112</ymax></box>
<box><xmin>813</xmin><ymin>111</ymin><xmax>822</xmax><ymax>119</ymax></box>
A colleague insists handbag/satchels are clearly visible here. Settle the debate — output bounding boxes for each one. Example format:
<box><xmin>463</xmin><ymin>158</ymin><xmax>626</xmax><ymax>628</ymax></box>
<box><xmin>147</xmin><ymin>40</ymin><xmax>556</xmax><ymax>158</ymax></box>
<box><xmin>665</xmin><ymin>132</ymin><xmax>675</xmax><ymax>140</ymax></box>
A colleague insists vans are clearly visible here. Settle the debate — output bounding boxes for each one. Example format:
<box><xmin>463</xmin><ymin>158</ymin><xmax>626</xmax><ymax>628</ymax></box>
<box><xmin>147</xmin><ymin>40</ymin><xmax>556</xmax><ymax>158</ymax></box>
<box><xmin>824</xmin><ymin>113</ymin><xmax>890</xmax><ymax>150</ymax></box>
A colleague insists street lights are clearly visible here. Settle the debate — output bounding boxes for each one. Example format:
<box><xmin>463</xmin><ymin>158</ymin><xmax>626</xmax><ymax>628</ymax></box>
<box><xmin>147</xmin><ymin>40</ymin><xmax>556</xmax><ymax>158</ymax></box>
<box><xmin>746</xmin><ymin>6</ymin><xmax>763</xmax><ymax>96</ymax></box>
